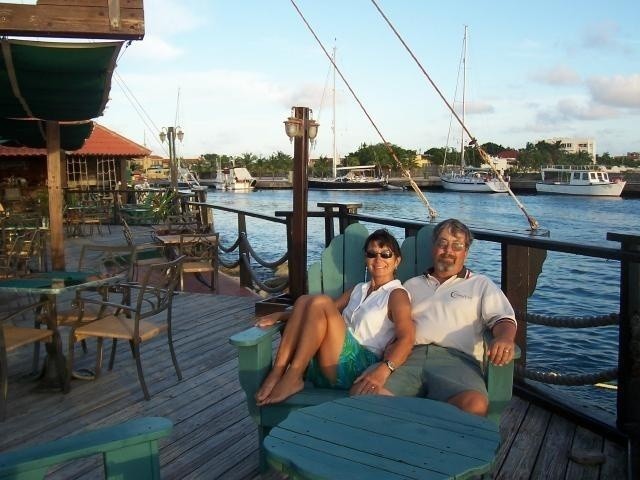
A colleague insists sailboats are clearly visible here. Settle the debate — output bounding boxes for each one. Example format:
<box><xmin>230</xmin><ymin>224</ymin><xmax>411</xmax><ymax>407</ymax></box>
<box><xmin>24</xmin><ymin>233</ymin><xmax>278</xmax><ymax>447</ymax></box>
<box><xmin>437</xmin><ymin>25</ymin><xmax>511</xmax><ymax>193</ymax></box>
<box><xmin>165</xmin><ymin>87</ymin><xmax>200</xmax><ymax>194</ymax></box>
<box><xmin>308</xmin><ymin>45</ymin><xmax>387</xmax><ymax>193</ymax></box>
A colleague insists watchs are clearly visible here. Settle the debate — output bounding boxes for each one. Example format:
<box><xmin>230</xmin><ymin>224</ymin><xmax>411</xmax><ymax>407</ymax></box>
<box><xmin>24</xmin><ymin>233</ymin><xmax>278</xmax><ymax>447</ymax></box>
<box><xmin>382</xmin><ymin>359</ymin><xmax>396</xmax><ymax>375</ymax></box>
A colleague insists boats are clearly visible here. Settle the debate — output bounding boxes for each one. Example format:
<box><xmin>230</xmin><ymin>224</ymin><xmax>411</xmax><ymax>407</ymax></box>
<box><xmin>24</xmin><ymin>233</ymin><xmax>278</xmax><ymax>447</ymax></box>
<box><xmin>535</xmin><ymin>165</ymin><xmax>627</xmax><ymax>197</ymax></box>
<box><xmin>212</xmin><ymin>154</ymin><xmax>256</xmax><ymax>190</ymax></box>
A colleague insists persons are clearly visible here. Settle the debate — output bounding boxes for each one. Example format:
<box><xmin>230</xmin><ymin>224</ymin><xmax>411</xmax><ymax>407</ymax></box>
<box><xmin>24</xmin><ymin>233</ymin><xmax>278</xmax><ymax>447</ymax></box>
<box><xmin>355</xmin><ymin>217</ymin><xmax>519</xmax><ymax>417</ymax></box>
<box><xmin>252</xmin><ymin>226</ymin><xmax>415</xmax><ymax>406</ymax></box>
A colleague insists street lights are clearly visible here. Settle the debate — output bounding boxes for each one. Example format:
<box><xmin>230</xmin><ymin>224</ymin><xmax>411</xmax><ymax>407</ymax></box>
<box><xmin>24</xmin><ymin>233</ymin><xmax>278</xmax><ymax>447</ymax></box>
<box><xmin>282</xmin><ymin>106</ymin><xmax>319</xmax><ymax>306</ymax></box>
<box><xmin>159</xmin><ymin>125</ymin><xmax>184</xmax><ymax>228</ymax></box>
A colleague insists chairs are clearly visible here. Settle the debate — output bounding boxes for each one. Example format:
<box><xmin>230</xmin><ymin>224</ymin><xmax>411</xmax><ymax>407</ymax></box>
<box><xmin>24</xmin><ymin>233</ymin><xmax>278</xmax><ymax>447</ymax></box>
<box><xmin>117</xmin><ymin>199</ymin><xmax>219</xmax><ymax>297</ymax></box>
<box><xmin>0</xmin><ymin>200</ymin><xmax>113</xmax><ymax>276</ymax></box>
<box><xmin>32</xmin><ymin>242</ymin><xmax>140</xmax><ymax>372</ymax></box>
<box><xmin>64</xmin><ymin>253</ymin><xmax>188</xmax><ymax>397</ymax></box>
<box><xmin>1</xmin><ymin>316</ymin><xmax>58</xmax><ymax>400</ymax></box>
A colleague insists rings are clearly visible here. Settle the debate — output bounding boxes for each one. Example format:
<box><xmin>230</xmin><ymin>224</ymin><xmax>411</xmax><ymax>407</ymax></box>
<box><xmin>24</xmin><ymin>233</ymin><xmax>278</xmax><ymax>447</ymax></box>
<box><xmin>371</xmin><ymin>386</ymin><xmax>375</xmax><ymax>389</ymax></box>
<box><xmin>504</xmin><ymin>349</ymin><xmax>509</xmax><ymax>353</ymax></box>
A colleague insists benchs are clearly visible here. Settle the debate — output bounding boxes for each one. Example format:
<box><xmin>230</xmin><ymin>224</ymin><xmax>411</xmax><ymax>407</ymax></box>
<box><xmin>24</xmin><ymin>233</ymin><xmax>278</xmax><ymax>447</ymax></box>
<box><xmin>229</xmin><ymin>223</ymin><xmax>521</xmax><ymax>474</ymax></box>
<box><xmin>0</xmin><ymin>417</ymin><xmax>173</xmax><ymax>480</ymax></box>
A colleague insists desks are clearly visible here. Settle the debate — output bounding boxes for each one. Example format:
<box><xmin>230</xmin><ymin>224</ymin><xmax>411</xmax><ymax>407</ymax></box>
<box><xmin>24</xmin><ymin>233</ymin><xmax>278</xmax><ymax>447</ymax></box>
<box><xmin>7</xmin><ymin>268</ymin><xmax>127</xmax><ymax>388</ymax></box>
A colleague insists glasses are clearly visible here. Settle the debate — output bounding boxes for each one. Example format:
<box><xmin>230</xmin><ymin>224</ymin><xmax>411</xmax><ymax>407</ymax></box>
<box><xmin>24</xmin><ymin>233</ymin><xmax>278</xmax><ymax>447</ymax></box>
<box><xmin>437</xmin><ymin>239</ymin><xmax>465</xmax><ymax>250</ymax></box>
<box><xmin>366</xmin><ymin>250</ymin><xmax>393</xmax><ymax>260</ymax></box>
